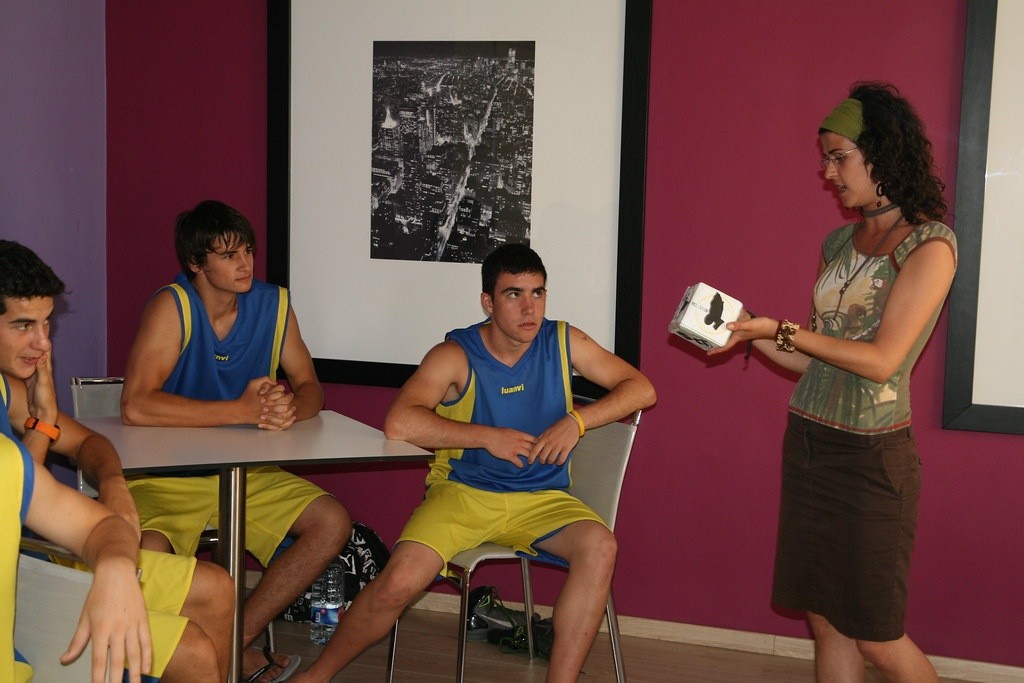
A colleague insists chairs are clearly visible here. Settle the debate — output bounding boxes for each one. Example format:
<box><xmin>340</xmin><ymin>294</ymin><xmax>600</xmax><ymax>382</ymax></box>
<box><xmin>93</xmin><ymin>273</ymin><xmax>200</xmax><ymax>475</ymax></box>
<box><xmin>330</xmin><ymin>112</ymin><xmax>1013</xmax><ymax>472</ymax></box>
<box><xmin>72</xmin><ymin>376</ymin><xmax>276</xmax><ymax>653</ymax></box>
<box><xmin>14</xmin><ymin>538</ymin><xmax>143</xmax><ymax>683</ymax></box>
<box><xmin>386</xmin><ymin>391</ymin><xmax>642</xmax><ymax>683</ymax></box>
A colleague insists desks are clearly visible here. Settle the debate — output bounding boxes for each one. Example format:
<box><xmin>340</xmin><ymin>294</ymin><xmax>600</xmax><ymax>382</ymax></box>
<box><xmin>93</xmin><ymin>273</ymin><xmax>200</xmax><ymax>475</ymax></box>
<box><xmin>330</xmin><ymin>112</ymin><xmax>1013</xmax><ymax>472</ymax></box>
<box><xmin>72</xmin><ymin>410</ymin><xmax>436</xmax><ymax>683</ymax></box>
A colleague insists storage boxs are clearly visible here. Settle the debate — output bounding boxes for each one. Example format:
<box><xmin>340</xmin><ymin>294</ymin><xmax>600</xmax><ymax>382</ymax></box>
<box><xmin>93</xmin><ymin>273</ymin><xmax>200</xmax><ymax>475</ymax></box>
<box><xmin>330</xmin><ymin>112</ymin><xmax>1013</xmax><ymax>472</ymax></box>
<box><xmin>667</xmin><ymin>281</ymin><xmax>743</xmax><ymax>352</ymax></box>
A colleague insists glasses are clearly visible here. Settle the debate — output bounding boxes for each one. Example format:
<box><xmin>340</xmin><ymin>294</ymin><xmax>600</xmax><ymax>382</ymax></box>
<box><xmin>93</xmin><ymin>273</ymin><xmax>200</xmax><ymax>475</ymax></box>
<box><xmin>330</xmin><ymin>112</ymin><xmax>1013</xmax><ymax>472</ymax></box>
<box><xmin>819</xmin><ymin>146</ymin><xmax>859</xmax><ymax>170</ymax></box>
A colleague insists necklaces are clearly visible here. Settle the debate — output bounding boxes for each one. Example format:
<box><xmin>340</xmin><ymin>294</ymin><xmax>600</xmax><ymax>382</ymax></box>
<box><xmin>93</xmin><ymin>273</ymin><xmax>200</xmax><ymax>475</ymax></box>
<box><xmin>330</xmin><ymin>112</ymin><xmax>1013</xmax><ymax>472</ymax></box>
<box><xmin>859</xmin><ymin>201</ymin><xmax>897</xmax><ymax>217</ymax></box>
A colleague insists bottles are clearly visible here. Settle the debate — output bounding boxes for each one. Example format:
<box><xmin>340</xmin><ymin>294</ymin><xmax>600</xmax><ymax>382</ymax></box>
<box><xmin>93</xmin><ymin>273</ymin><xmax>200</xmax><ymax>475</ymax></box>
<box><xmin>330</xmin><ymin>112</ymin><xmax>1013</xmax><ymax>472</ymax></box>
<box><xmin>310</xmin><ymin>559</ymin><xmax>346</xmax><ymax>645</ymax></box>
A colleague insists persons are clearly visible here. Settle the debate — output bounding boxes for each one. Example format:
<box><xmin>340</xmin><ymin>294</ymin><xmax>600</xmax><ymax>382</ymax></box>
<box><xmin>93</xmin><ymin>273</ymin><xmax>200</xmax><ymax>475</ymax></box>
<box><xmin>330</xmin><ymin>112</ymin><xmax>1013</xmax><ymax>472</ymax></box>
<box><xmin>121</xmin><ymin>202</ymin><xmax>350</xmax><ymax>646</ymax></box>
<box><xmin>0</xmin><ymin>432</ymin><xmax>153</xmax><ymax>683</ymax></box>
<box><xmin>706</xmin><ymin>80</ymin><xmax>958</xmax><ymax>683</ymax></box>
<box><xmin>287</xmin><ymin>245</ymin><xmax>656</xmax><ymax>682</ymax></box>
<box><xmin>0</xmin><ymin>239</ymin><xmax>304</xmax><ymax>683</ymax></box>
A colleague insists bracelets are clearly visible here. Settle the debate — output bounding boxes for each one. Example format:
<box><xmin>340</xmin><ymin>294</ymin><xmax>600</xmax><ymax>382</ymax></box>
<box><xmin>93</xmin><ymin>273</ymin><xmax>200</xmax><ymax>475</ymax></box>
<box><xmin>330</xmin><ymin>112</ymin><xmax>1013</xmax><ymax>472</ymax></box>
<box><xmin>775</xmin><ymin>319</ymin><xmax>800</xmax><ymax>352</ymax></box>
<box><xmin>24</xmin><ymin>416</ymin><xmax>62</xmax><ymax>446</ymax></box>
<box><xmin>744</xmin><ymin>311</ymin><xmax>757</xmax><ymax>363</ymax></box>
<box><xmin>571</xmin><ymin>410</ymin><xmax>585</xmax><ymax>436</ymax></box>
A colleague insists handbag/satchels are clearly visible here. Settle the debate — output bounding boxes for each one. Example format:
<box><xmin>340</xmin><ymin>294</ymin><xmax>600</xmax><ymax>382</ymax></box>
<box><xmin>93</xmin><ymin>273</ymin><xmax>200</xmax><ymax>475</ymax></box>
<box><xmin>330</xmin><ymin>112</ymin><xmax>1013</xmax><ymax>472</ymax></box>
<box><xmin>278</xmin><ymin>521</ymin><xmax>391</xmax><ymax>622</ymax></box>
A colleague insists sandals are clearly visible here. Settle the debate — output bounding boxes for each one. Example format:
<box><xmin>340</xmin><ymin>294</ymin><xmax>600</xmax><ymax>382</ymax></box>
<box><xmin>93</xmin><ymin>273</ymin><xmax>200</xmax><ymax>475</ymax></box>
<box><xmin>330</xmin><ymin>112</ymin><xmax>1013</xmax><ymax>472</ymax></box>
<box><xmin>245</xmin><ymin>649</ymin><xmax>301</xmax><ymax>683</ymax></box>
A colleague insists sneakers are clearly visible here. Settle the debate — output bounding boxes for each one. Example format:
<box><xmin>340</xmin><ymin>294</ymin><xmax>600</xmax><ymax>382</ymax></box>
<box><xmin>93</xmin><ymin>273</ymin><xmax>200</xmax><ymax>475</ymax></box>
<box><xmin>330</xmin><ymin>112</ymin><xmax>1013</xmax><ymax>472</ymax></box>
<box><xmin>465</xmin><ymin>586</ymin><xmax>541</xmax><ymax>643</ymax></box>
<box><xmin>488</xmin><ymin>617</ymin><xmax>554</xmax><ymax>662</ymax></box>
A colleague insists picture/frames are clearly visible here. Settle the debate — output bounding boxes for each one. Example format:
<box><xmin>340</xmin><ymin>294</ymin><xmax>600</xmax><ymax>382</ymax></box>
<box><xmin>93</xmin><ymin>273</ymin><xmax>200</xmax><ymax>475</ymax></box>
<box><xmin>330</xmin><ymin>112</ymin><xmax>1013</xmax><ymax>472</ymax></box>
<box><xmin>940</xmin><ymin>0</ymin><xmax>1024</xmax><ymax>436</ymax></box>
<box><xmin>264</xmin><ymin>0</ymin><xmax>653</xmax><ymax>401</ymax></box>
